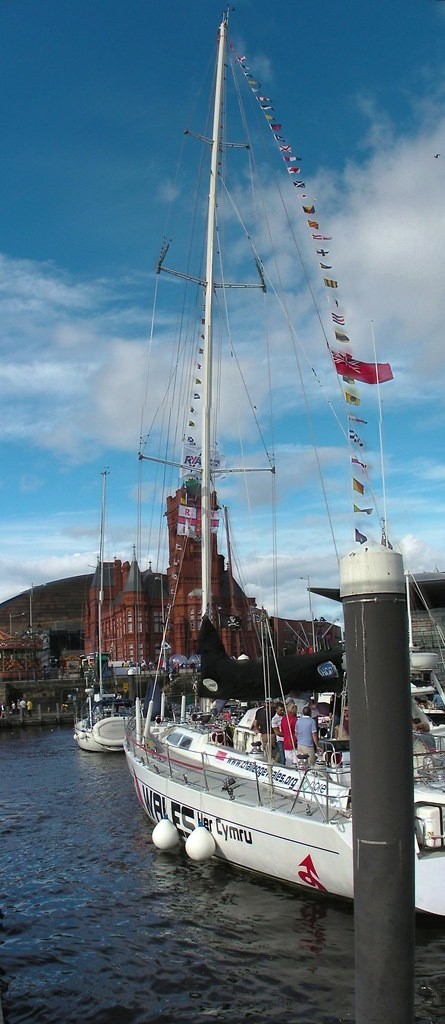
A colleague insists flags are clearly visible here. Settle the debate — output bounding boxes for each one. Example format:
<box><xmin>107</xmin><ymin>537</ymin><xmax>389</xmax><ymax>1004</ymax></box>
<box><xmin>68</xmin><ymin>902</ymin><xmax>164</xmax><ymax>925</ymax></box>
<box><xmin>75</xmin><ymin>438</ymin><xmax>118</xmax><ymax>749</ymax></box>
<box><xmin>176</xmin><ymin>498</ymin><xmax>222</xmax><ymax>541</ymax></box>
<box><xmin>236</xmin><ymin>52</ymin><xmax>333</xmax><ymax>270</ymax></box>
<box><xmin>324</xmin><ymin>276</ymin><xmax>349</xmax><ymax>344</ymax></box>
<box><xmin>345</xmin><ymin>389</ymin><xmax>373</xmax><ymax>544</ymax></box>
<box><xmin>187</xmin><ymin>318</ymin><xmax>206</xmax><ymax>427</ymax></box>
<box><xmin>330</xmin><ymin>347</ymin><xmax>394</xmax><ymax>385</ymax></box>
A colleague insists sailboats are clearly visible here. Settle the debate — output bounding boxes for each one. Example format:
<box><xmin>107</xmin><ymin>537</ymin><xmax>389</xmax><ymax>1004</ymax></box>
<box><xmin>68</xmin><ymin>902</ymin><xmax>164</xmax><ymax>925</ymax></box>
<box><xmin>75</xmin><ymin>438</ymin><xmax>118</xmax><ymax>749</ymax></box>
<box><xmin>71</xmin><ymin>14</ymin><xmax>444</xmax><ymax>921</ymax></box>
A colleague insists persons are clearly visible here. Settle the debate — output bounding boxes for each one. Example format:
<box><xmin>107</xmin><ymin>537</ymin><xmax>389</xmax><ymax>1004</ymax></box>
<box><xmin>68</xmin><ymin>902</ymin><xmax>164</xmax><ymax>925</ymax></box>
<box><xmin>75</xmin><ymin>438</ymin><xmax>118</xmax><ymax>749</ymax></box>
<box><xmin>153</xmin><ymin>709</ymin><xmax>245</xmax><ymax>748</ymax></box>
<box><xmin>246</xmin><ymin>688</ymin><xmax>350</xmax><ymax>768</ymax></box>
<box><xmin>412</xmin><ymin>690</ymin><xmax>445</xmax><ymax>767</ymax></box>
<box><xmin>121</xmin><ymin>659</ymin><xmax>185</xmax><ymax>675</ymax></box>
<box><xmin>238</xmin><ymin>651</ymin><xmax>249</xmax><ymax>660</ymax></box>
<box><xmin>0</xmin><ymin>698</ymin><xmax>34</xmax><ymax>719</ymax></box>
